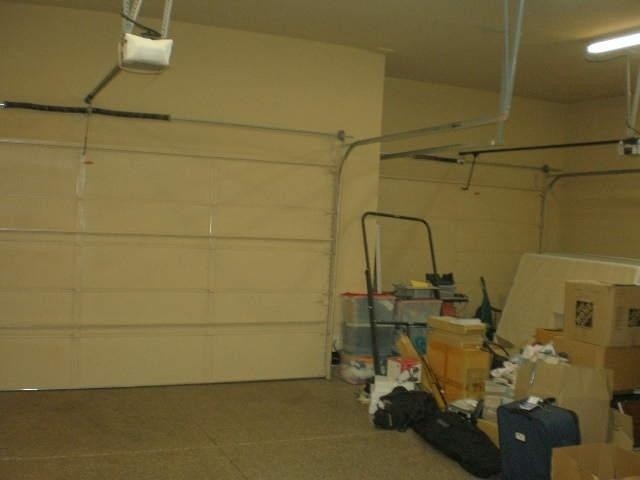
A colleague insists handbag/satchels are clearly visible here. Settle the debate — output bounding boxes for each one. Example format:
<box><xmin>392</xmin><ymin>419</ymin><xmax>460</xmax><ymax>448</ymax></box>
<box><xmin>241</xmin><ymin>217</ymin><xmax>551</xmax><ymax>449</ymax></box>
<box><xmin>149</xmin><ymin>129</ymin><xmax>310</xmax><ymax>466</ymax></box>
<box><xmin>414</xmin><ymin>410</ymin><xmax>502</xmax><ymax>478</ymax></box>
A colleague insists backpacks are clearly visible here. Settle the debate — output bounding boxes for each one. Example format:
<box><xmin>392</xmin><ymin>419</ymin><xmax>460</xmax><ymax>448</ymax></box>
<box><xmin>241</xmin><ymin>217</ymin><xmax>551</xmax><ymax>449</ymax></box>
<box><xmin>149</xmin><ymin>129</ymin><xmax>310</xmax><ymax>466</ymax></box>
<box><xmin>373</xmin><ymin>386</ymin><xmax>438</xmax><ymax>431</ymax></box>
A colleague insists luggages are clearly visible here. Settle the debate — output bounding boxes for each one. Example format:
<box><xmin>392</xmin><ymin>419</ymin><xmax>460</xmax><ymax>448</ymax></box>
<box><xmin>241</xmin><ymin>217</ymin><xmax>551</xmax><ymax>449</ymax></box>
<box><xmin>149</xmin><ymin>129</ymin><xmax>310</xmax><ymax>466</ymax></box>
<box><xmin>497</xmin><ymin>397</ymin><xmax>581</xmax><ymax>479</ymax></box>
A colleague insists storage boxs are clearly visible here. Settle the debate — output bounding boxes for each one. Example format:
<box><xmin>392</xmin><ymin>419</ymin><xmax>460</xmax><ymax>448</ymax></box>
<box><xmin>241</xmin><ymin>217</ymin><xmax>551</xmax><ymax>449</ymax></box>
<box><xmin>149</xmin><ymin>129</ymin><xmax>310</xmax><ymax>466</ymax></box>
<box><xmin>336</xmin><ymin>280</ymin><xmax>640</xmax><ymax>480</ymax></box>
